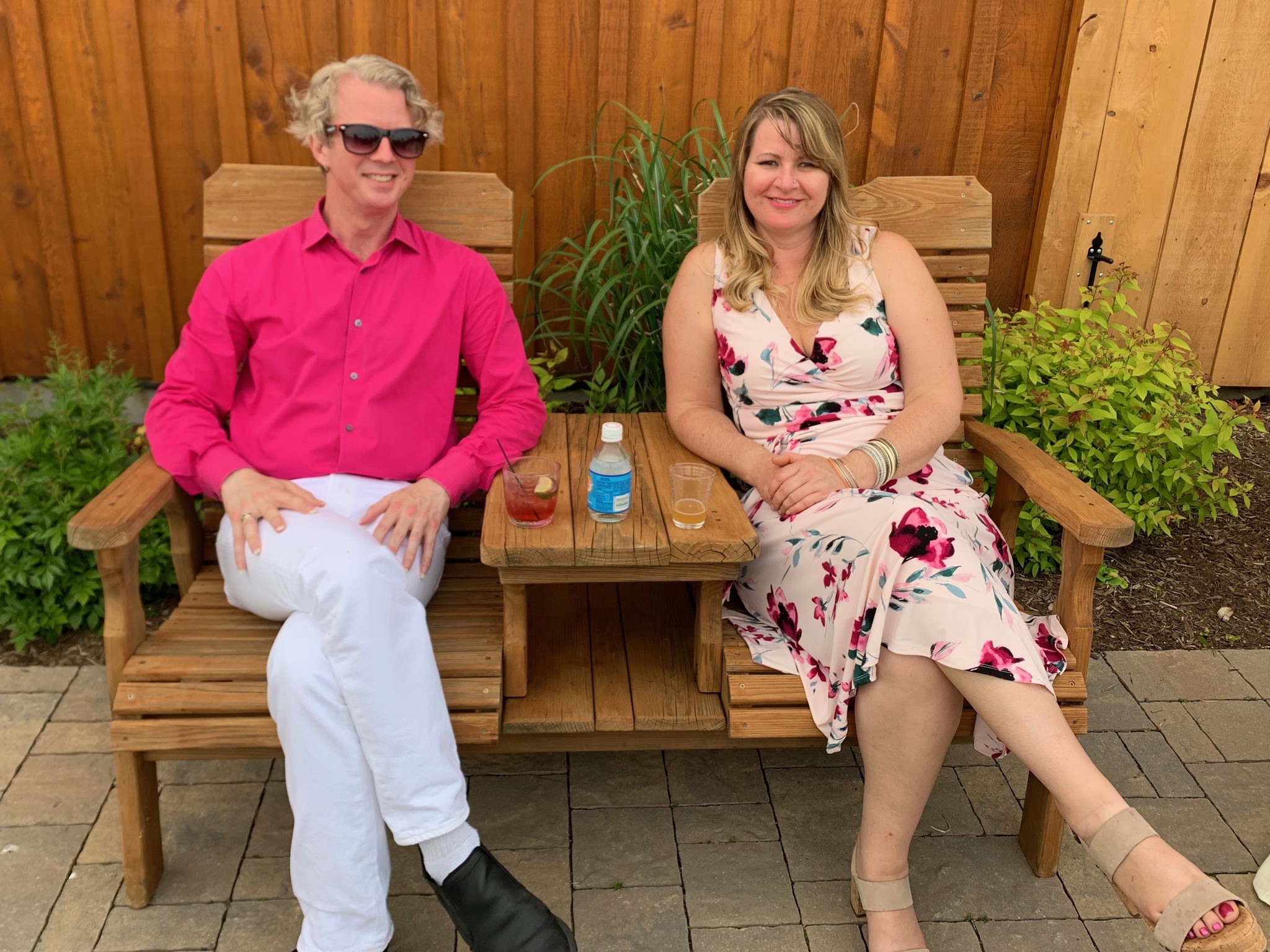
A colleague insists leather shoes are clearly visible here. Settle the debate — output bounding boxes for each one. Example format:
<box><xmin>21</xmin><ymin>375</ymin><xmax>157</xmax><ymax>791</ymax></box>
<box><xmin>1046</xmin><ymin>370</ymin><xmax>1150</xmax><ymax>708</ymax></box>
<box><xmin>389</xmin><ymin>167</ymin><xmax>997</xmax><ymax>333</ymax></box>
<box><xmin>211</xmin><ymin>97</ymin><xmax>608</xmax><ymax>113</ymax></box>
<box><xmin>417</xmin><ymin>840</ymin><xmax>578</xmax><ymax>952</ymax></box>
<box><xmin>291</xmin><ymin>944</ymin><xmax>389</xmax><ymax>952</ymax></box>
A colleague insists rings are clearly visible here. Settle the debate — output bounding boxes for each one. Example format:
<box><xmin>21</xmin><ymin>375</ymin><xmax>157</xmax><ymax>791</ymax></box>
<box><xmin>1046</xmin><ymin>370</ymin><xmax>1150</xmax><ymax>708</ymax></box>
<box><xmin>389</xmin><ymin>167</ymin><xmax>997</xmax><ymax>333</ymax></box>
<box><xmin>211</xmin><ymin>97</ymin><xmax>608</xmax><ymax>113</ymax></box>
<box><xmin>241</xmin><ymin>512</ymin><xmax>251</xmax><ymax>523</ymax></box>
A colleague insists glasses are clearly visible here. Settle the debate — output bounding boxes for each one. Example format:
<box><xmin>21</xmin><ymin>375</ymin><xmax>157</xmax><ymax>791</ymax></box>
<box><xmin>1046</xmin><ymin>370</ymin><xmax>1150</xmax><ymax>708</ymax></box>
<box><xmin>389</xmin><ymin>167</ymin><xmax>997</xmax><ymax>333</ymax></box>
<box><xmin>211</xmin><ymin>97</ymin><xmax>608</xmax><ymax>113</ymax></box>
<box><xmin>325</xmin><ymin>124</ymin><xmax>430</xmax><ymax>159</ymax></box>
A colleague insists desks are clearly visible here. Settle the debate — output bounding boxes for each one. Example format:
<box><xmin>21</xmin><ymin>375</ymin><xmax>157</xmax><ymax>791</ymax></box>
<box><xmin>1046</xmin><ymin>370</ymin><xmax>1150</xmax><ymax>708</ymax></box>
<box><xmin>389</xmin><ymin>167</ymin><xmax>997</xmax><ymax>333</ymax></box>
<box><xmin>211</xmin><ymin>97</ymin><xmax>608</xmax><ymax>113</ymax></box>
<box><xmin>482</xmin><ymin>413</ymin><xmax>758</xmax><ymax>697</ymax></box>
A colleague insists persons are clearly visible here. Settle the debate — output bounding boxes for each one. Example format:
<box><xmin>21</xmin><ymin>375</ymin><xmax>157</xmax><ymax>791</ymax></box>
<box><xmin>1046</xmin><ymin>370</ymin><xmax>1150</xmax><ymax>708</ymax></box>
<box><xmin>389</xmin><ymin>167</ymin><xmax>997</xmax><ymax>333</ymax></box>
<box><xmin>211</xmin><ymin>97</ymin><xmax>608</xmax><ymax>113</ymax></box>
<box><xmin>661</xmin><ymin>86</ymin><xmax>1269</xmax><ymax>952</ymax></box>
<box><xmin>141</xmin><ymin>52</ymin><xmax>579</xmax><ymax>952</ymax></box>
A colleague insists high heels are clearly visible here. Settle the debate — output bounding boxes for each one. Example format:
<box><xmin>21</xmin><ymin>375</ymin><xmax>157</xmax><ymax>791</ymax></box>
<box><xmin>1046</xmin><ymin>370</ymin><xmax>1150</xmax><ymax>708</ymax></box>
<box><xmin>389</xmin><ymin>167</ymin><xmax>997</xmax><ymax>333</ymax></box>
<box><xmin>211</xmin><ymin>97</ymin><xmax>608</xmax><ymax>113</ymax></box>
<box><xmin>851</xmin><ymin>840</ymin><xmax>930</xmax><ymax>952</ymax></box>
<box><xmin>1063</xmin><ymin>807</ymin><xmax>1267</xmax><ymax>952</ymax></box>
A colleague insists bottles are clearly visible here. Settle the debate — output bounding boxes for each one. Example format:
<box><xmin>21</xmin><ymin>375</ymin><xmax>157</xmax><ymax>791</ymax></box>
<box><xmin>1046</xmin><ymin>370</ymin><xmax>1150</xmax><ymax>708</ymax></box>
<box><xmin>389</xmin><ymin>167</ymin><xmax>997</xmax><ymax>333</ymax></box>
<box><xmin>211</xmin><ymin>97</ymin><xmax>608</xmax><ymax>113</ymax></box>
<box><xmin>588</xmin><ymin>422</ymin><xmax>632</xmax><ymax>522</ymax></box>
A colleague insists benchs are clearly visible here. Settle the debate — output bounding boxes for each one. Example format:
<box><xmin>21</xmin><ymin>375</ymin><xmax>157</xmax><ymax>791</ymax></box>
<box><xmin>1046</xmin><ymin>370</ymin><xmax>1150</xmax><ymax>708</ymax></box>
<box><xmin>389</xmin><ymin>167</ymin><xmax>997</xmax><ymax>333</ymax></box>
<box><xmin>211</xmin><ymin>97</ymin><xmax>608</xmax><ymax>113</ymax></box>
<box><xmin>66</xmin><ymin>163</ymin><xmax>1136</xmax><ymax>909</ymax></box>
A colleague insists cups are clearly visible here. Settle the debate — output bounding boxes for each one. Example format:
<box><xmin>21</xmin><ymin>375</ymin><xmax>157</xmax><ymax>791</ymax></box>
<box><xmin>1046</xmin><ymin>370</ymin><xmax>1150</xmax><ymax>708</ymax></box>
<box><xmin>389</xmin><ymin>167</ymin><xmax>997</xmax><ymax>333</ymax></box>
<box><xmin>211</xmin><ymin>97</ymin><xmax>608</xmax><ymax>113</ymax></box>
<box><xmin>668</xmin><ymin>463</ymin><xmax>716</xmax><ymax>530</ymax></box>
<box><xmin>502</xmin><ymin>456</ymin><xmax>560</xmax><ymax>528</ymax></box>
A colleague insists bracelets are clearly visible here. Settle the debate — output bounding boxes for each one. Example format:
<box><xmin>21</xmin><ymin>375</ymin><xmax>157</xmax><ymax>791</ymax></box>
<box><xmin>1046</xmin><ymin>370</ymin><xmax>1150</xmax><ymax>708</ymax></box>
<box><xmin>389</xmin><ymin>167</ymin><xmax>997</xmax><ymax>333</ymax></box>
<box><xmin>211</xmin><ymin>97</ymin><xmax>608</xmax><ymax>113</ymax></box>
<box><xmin>849</xmin><ymin>436</ymin><xmax>899</xmax><ymax>489</ymax></box>
<box><xmin>829</xmin><ymin>456</ymin><xmax>860</xmax><ymax>489</ymax></box>
